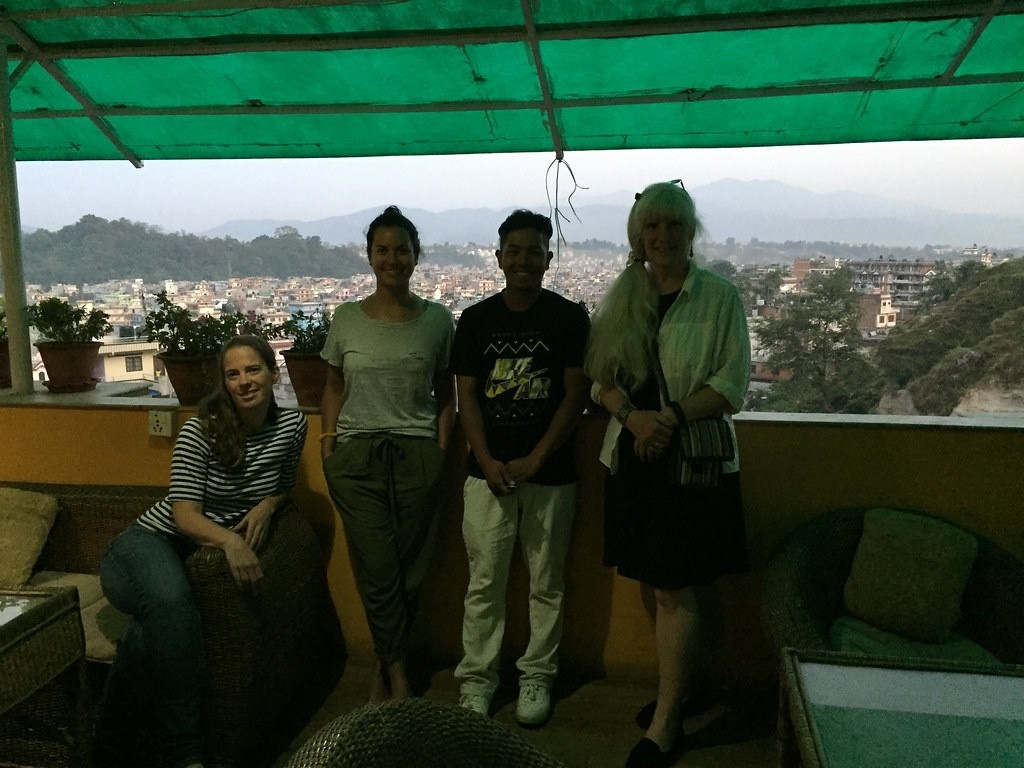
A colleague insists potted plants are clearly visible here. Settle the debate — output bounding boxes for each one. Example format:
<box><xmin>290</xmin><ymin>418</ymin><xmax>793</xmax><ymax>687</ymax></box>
<box><xmin>135</xmin><ymin>290</ymin><xmax>249</xmax><ymax>404</ymax></box>
<box><xmin>20</xmin><ymin>294</ymin><xmax>114</xmax><ymax>392</ymax></box>
<box><xmin>0</xmin><ymin>309</ymin><xmax>11</xmax><ymax>388</ymax></box>
<box><xmin>245</xmin><ymin>307</ymin><xmax>335</xmax><ymax>407</ymax></box>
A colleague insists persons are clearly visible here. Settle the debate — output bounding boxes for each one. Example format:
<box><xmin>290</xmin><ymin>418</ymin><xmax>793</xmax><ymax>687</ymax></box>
<box><xmin>93</xmin><ymin>336</ymin><xmax>307</xmax><ymax>768</ymax></box>
<box><xmin>586</xmin><ymin>178</ymin><xmax>752</xmax><ymax>768</ymax></box>
<box><xmin>318</xmin><ymin>207</ymin><xmax>455</xmax><ymax>701</ymax></box>
<box><xmin>454</xmin><ymin>211</ymin><xmax>593</xmax><ymax>725</ymax></box>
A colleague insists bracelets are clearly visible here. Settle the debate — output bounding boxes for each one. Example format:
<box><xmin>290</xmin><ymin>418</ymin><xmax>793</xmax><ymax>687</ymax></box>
<box><xmin>667</xmin><ymin>401</ymin><xmax>685</xmax><ymax>423</ymax></box>
<box><xmin>617</xmin><ymin>402</ymin><xmax>637</xmax><ymax>426</ymax></box>
<box><xmin>319</xmin><ymin>433</ymin><xmax>337</xmax><ymax>441</ymax></box>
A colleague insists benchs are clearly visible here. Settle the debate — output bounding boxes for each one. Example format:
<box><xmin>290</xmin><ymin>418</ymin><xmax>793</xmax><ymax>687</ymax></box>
<box><xmin>0</xmin><ymin>481</ymin><xmax>323</xmax><ymax>768</ymax></box>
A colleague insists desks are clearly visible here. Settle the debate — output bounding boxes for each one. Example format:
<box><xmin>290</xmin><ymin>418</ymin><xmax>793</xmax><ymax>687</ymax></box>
<box><xmin>769</xmin><ymin>644</ymin><xmax>1024</xmax><ymax>766</ymax></box>
<box><xmin>0</xmin><ymin>586</ymin><xmax>87</xmax><ymax>715</ymax></box>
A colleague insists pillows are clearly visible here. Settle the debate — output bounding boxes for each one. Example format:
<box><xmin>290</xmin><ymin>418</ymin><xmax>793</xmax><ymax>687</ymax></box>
<box><xmin>0</xmin><ymin>488</ymin><xmax>59</xmax><ymax>587</ymax></box>
<box><xmin>841</xmin><ymin>507</ymin><xmax>977</xmax><ymax>643</ymax></box>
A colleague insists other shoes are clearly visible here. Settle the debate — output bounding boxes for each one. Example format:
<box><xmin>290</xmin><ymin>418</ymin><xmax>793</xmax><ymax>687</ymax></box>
<box><xmin>626</xmin><ymin>726</ymin><xmax>686</xmax><ymax>768</ymax></box>
<box><xmin>635</xmin><ymin>689</ymin><xmax>707</xmax><ymax>728</ymax></box>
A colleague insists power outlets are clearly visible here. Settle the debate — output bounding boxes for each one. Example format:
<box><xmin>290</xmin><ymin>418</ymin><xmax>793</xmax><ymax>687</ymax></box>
<box><xmin>148</xmin><ymin>410</ymin><xmax>178</xmax><ymax>437</ymax></box>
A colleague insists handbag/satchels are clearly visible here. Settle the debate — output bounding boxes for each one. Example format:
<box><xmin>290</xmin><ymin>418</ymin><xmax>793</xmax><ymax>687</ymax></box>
<box><xmin>674</xmin><ymin>417</ymin><xmax>734</xmax><ymax>491</ymax></box>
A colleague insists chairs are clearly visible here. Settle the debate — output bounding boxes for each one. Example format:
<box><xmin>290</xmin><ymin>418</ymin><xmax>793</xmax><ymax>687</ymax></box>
<box><xmin>752</xmin><ymin>502</ymin><xmax>1023</xmax><ymax>671</ymax></box>
<box><xmin>284</xmin><ymin>697</ymin><xmax>567</xmax><ymax>767</ymax></box>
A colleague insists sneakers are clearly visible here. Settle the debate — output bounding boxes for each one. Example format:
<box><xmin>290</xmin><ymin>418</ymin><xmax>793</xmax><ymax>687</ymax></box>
<box><xmin>457</xmin><ymin>692</ymin><xmax>491</xmax><ymax>719</ymax></box>
<box><xmin>516</xmin><ymin>685</ymin><xmax>551</xmax><ymax>724</ymax></box>
